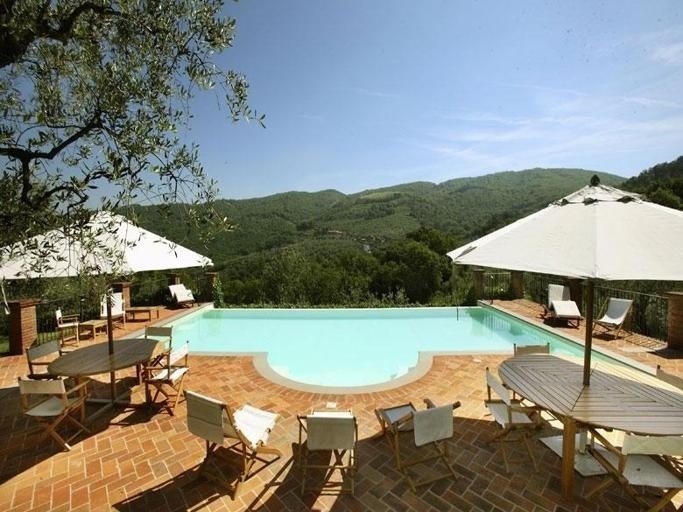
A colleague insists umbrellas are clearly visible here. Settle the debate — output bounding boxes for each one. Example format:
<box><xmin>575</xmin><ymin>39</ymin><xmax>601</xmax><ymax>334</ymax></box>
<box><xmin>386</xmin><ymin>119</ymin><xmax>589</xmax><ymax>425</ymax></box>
<box><xmin>445</xmin><ymin>172</ymin><xmax>682</xmax><ymax>455</ymax></box>
<box><xmin>0</xmin><ymin>210</ymin><xmax>214</xmax><ymax>401</ymax></box>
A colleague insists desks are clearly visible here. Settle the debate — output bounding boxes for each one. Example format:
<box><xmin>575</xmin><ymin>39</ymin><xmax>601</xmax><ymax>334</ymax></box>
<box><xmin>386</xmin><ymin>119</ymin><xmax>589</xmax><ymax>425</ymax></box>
<box><xmin>79</xmin><ymin>320</ymin><xmax>107</xmax><ymax>339</ymax></box>
<box><xmin>125</xmin><ymin>306</ymin><xmax>165</xmax><ymax>322</ymax></box>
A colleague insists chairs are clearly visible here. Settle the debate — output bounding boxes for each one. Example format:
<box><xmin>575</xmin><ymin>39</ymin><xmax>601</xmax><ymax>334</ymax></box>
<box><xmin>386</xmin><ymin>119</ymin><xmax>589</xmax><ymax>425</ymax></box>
<box><xmin>166</xmin><ymin>283</ymin><xmax>195</xmax><ymax>309</ymax></box>
<box><xmin>482</xmin><ymin>343</ymin><xmax>683</xmax><ymax>512</ymax></box>
<box><xmin>18</xmin><ymin>325</ymin><xmax>188</xmax><ymax>451</ymax></box>
<box><xmin>182</xmin><ymin>388</ymin><xmax>461</xmax><ymax>500</ymax></box>
<box><xmin>538</xmin><ymin>284</ymin><xmax>634</xmax><ymax>340</ymax></box>
<box><xmin>56</xmin><ymin>309</ymin><xmax>78</xmax><ymax>348</ymax></box>
